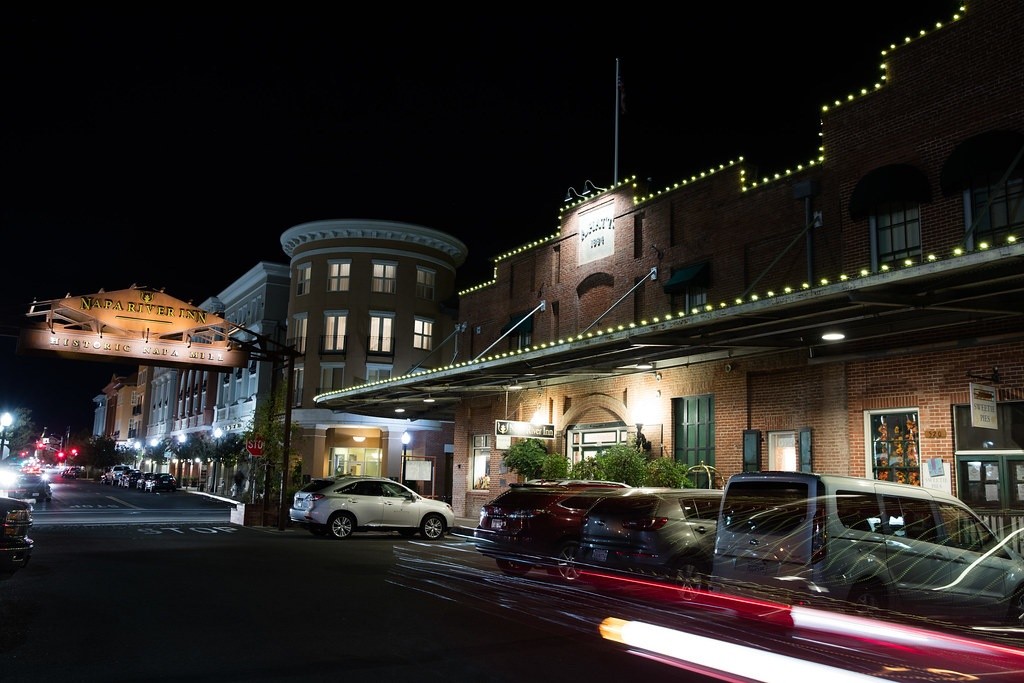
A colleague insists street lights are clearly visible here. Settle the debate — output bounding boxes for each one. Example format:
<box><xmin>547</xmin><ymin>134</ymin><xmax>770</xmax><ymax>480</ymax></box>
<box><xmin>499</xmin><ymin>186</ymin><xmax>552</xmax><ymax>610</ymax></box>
<box><xmin>210</xmin><ymin>427</ymin><xmax>224</xmax><ymax>492</ymax></box>
<box><xmin>401</xmin><ymin>432</ymin><xmax>411</xmax><ymax>486</ymax></box>
<box><xmin>0</xmin><ymin>412</ymin><xmax>12</xmax><ymax>463</ymax></box>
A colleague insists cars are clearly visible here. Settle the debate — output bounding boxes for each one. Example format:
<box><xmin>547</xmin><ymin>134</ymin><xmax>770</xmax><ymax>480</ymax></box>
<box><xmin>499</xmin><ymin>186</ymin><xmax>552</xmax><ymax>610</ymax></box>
<box><xmin>0</xmin><ymin>441</ymin><xmax>180</xmax><ymax>585</ymax></box>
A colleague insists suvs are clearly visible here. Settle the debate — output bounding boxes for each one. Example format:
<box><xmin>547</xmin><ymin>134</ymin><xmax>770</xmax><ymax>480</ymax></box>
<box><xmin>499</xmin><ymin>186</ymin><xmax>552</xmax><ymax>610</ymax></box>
<box><xmin>467</xmin><ymin>479</ymin><xmax>630</xmax><ymax>583</ymax></box>
<box><xmin>707</xmin><ymin>471</ymin><xmax>1024</xmax><ymax>632</ymax></box>
<box><xmin>575</xmin><ymin>487</ymin><xmax>725</xmax><ymax>598</ymax></box>
<box><xmin>290</xmin><ymin>473</ymin><xmax>457</xmax><ymax>542</ymax></box>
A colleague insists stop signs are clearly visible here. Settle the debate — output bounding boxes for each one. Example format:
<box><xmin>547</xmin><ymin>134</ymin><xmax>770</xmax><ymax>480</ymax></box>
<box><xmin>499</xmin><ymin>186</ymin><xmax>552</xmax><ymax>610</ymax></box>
<box><xmin>245</xmin><ymin>432</ymin><xmax>269</xmax><ymax>456</ymax></box>
